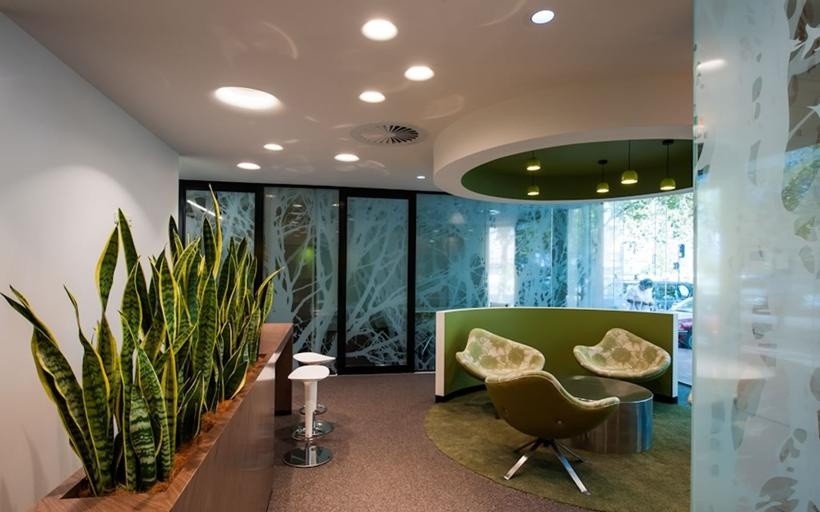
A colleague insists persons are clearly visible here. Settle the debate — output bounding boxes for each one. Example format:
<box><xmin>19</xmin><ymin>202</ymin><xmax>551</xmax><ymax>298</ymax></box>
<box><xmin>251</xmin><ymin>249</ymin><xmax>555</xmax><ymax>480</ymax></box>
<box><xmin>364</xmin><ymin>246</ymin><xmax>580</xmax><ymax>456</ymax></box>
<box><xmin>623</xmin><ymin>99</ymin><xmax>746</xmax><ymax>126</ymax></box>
<box><xmin>626</xmin><ymin>277</ymin><xmax>656</xmax><ymax>312</ymax></box>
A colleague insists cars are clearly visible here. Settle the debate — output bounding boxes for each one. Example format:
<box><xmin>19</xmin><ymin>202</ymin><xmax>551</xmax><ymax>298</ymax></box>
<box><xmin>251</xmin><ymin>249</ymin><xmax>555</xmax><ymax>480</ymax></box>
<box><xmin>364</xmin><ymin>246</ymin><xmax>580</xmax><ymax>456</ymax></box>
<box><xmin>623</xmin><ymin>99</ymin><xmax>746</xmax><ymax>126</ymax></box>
<box><xmin>666</xmin><ymin>298</ymin><xmax>692</xmax><ymax>348</ymax></box>
<box><xmin>603</xmin><ymin>279</ymin><xmax>692</xmax><ymax>314</ymax></box>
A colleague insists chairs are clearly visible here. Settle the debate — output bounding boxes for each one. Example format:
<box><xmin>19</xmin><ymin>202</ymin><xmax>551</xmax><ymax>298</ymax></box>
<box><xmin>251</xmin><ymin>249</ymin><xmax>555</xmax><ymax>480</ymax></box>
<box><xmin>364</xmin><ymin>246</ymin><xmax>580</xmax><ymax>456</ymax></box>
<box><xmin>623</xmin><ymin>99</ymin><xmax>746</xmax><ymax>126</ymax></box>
<box><xmin>455</xmin><ymin>327</ymin><xmax>672</xmax><ymax>495</ymax></box>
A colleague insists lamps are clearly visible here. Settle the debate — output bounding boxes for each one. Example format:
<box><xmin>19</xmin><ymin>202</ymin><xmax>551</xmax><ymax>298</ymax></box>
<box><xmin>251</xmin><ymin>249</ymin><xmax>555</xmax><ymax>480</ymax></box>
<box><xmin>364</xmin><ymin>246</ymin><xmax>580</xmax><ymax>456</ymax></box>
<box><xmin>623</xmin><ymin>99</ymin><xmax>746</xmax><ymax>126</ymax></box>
<box><xmin>524</xmin><ymin>138</ymin><xmax>677</xmax><ymax>196</ymax></box>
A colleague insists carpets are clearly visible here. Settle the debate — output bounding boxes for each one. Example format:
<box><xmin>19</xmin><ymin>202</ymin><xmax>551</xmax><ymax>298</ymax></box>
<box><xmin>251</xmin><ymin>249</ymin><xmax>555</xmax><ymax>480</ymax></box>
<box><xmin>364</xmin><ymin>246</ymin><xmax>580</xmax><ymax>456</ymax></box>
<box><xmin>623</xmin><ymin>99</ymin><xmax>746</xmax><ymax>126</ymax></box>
<box><xmin>423</xmin><ymin>387</ymin><xmax>691</xmax><ymax>512</ymax></box>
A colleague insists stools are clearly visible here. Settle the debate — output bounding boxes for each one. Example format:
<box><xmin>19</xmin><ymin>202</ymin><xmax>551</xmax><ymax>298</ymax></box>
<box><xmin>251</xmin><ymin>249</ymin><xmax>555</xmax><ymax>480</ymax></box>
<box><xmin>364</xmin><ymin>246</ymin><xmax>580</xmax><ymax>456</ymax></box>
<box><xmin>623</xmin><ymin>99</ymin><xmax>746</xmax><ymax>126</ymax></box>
<box><xmin>280</xmin><ymin>351</ymin><xmax>337</xmax><ymax>469</ymax></box>
